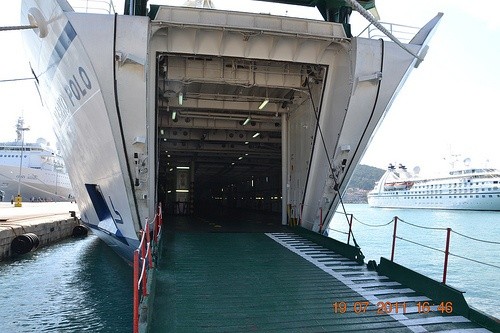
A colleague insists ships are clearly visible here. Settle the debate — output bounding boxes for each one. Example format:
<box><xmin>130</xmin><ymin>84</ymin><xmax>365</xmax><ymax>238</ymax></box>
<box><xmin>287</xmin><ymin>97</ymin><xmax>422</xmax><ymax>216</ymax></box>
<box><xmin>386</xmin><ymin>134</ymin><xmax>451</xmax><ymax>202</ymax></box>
<box><xmin>366</xmin><ymin>155</ymin><xmax>499</xmax><ymax>210</ymax></box>
<box><xmin>1</xmin><ymin>116</ymin><xmax>75</xmax><ymax>202</ymax></box>
<box><xmin>18</xmin><ymin>0</ymin><xmax>499</xmax><ymax>333</ymax></box>
